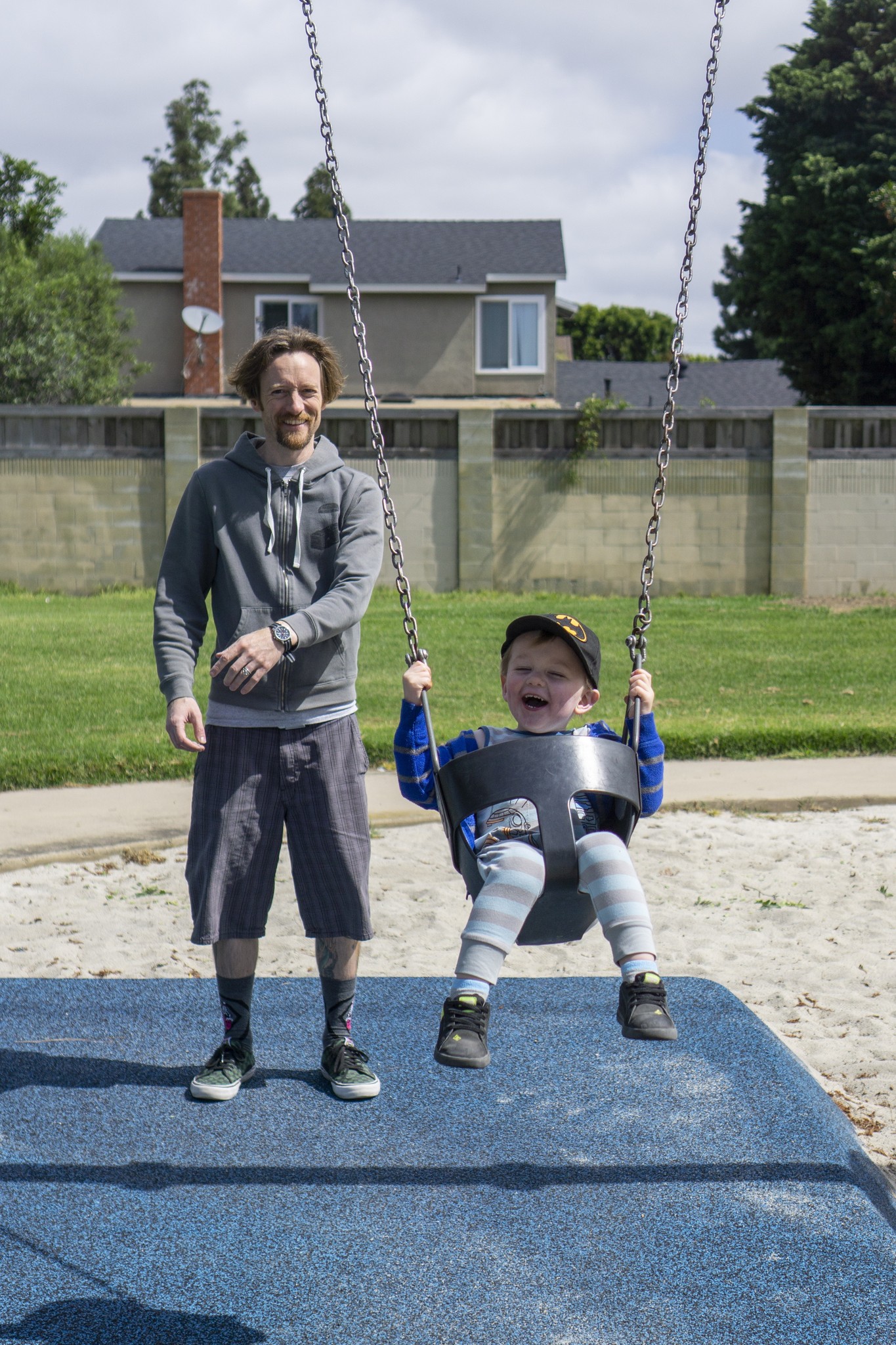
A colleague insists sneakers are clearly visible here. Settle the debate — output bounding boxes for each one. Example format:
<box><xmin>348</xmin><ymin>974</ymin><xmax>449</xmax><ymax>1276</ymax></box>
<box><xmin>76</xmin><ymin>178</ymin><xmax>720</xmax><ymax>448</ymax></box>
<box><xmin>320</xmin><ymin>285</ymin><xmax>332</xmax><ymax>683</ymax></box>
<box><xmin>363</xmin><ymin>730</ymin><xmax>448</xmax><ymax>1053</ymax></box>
<box><xmin>432</xmin><ymin>994</ymin><xmax>492</xmax><ymax>1068</ymax></box>
<box><xmin>190</xmin><ymin>1037</ymin><xmax>257</xmax><ymax>1101</ymax></box>
<box><xmin>616</xmin><ymin>971</ymin><xmax>679</xmax><ymax>1041</ymax></box>
<box><xmin>321</xmin><ymin>1038</ymin><xmax>381</xmax><ymax>1099</ymax></box>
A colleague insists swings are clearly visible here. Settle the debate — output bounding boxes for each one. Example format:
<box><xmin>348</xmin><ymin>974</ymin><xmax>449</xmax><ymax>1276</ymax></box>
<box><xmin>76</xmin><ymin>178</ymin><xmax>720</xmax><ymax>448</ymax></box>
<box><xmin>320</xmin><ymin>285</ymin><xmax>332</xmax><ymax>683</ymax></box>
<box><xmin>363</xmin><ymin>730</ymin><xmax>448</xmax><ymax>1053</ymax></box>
<box><xmin>301</xmin><ymin>0</ymin><xmax>736</xmax><ymax>949</ymax></box>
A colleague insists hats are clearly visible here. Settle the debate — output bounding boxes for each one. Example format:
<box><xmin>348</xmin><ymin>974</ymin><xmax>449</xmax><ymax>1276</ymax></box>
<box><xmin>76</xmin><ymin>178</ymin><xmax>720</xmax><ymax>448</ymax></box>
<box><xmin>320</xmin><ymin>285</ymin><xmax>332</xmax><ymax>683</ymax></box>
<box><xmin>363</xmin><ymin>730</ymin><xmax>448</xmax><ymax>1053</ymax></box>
<box><xmin>500</xmin><ymin>614</ymin><xmax>601</xmax><ymax>689</ymax></box>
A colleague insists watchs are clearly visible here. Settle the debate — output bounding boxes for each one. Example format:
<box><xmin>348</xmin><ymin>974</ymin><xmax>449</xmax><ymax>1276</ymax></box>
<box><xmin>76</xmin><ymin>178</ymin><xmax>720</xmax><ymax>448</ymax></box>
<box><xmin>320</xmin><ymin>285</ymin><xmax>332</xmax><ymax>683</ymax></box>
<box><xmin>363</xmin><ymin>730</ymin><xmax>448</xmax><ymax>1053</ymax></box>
<box><xmin>268</xmin><ymin>622</ymin><xmax>292</xmax><ymax>652</ymax></box>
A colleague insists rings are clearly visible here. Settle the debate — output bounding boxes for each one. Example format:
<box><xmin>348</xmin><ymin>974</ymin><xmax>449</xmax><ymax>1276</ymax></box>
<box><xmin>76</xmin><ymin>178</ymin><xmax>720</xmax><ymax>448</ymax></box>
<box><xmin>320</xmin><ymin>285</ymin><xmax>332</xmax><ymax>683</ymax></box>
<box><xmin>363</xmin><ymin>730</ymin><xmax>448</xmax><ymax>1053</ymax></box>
<box><xmin>242</xmin><ymin>667</ymin><xmax>252</xmax><ymax>675</ymax></box>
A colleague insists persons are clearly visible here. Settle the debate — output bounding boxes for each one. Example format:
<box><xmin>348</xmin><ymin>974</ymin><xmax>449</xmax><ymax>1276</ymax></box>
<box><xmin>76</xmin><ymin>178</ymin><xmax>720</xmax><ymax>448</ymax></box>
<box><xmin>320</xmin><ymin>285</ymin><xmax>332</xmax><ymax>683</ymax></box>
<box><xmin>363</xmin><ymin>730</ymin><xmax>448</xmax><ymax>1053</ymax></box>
<box><xmin>394</xmin><ymin>615</ymin><xmax>678</xmax><ymax>1065</ymax></box>
<box><xmin>153</xmin><ymin>326</ymin><xmax>387</xmax><ymax>1107</ymax></box>
